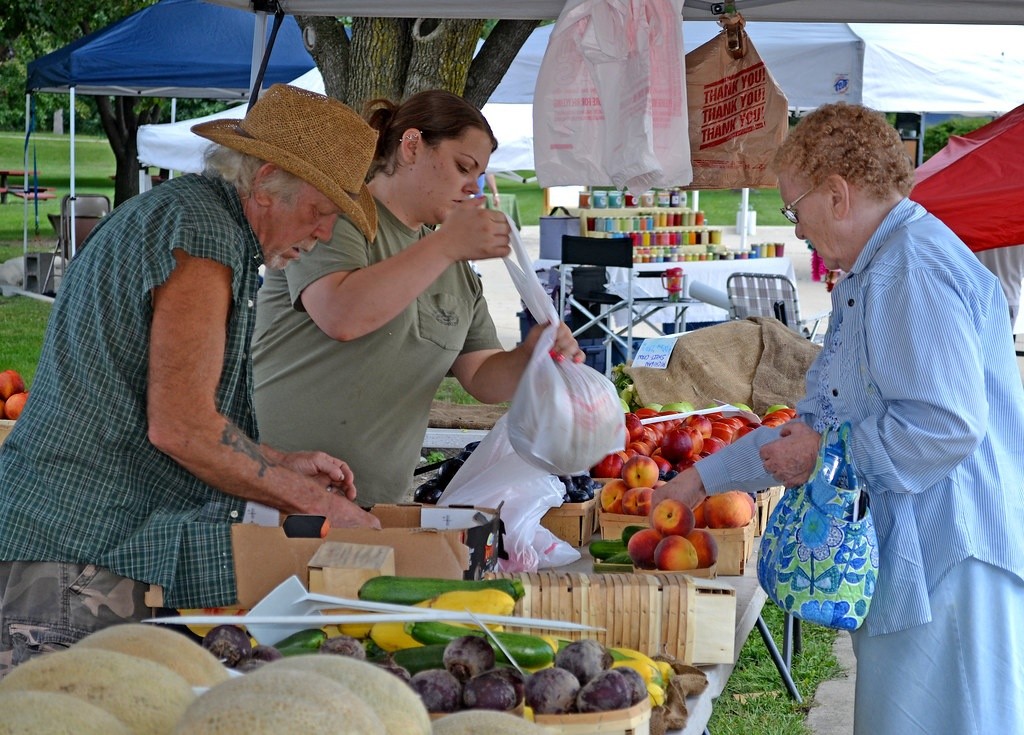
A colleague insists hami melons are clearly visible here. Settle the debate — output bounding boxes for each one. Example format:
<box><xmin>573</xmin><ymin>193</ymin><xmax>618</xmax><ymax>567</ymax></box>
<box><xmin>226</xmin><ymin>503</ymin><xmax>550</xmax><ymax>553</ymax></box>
<box><xmin>0</xmin><ymin>624</ymin><xmax>569</xmax><ymax>735</ymax></box>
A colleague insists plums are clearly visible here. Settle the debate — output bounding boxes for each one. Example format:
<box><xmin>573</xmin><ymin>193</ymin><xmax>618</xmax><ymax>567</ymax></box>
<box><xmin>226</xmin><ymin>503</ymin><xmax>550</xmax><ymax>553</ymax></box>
<box><xmin>412</xmin><ymin>441</ymin><xmax>601</xmax><ymax>504</ymax></box>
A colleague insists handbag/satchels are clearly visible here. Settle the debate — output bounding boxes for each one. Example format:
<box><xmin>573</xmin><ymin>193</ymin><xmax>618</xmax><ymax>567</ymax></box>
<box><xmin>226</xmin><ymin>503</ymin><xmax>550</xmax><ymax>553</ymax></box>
<box><xmin>757</xmin><ymin>421</ymin><xmax>881</xmax><ymax>630</ymax></box>
<box><xmin>493</xmin><ymin>211</ymin><xmax>626</xmax><ymax>477</ymax></box>
<box><xmin>439</xmin><ymin>412</ymin><xmax>581</xmax><ymax>576</ymax></box>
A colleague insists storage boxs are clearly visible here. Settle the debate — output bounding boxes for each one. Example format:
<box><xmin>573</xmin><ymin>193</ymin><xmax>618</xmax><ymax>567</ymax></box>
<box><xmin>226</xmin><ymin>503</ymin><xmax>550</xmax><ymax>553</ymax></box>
<box><xmin>230</xmin><ymin>408</ymin><xmax>789</xmax><ymax>672</ymax></box>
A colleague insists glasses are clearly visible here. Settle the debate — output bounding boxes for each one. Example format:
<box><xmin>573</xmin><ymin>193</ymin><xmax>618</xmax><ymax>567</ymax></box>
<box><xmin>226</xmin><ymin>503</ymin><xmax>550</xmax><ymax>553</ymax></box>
<box><xmin>781</xmin><ymin>171</ymin><xmax>851</xmax><ymax>223</ymax></box>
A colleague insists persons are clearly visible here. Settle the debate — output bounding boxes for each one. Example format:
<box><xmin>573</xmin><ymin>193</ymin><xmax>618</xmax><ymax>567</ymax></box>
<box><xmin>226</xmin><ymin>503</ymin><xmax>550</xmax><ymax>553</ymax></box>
<box><xmin>475</xmin><ymin>174</ymin><xmax>499</xmax><ymax>207</ymax></box>
<box><xmin>975</xmin><ymin>245</ymin><xmax>1024</xmax><ymax>329</ymax></box>
<box><xmin>654</xmin><ymin>102</ymin><xmax>1024</xmax><ymax>735</ymax></box>
<box><xmin>245</xmin><ymin>91</ymin><xmax>586</xmax><ymax>526</ymax></box>
<box><xmin>1</xmin><ymin>85</ymin><xmax>380</xmax><ymax>677</ymax></box>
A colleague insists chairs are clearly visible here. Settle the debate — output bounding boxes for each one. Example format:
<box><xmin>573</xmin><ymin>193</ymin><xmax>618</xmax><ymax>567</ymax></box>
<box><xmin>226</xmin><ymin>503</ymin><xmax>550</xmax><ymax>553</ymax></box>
<box><xmin>559</xmin><ymin>234</ymin><xmax>829</xmax><ymax>383</ymax></box>
<box><xmin>42</xmin><ymin>192</ymin><xmax>110</xmax><ymax>293</ymax></box>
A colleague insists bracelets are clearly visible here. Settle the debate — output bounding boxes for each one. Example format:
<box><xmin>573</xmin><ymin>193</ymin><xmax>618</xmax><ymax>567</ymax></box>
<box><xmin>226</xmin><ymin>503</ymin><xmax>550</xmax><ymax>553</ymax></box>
<box><xmin>493</xmin><ymin>194</ymin><xmax>498</xmax><ymax>196</ymax></box>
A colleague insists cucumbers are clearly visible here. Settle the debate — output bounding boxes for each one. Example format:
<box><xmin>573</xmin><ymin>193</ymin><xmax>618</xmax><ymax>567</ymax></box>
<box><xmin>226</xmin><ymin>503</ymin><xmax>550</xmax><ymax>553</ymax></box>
<box><xmin>589</xmin><ymin>525</ymin><xmax>648</xmax><ymax>566</ymax></box>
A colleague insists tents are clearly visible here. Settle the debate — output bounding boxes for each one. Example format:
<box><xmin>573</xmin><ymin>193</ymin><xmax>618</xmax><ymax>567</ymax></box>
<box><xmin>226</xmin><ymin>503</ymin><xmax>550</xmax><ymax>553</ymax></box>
<box><xmin>24</xmin><ymin>1</ymin><xmax>350</xmax><ymax>257</ymax></box>
<box><xmin>139</xmin><ymin>0</ymin><xmax>1024</xmax><ymax>259</ymax></box>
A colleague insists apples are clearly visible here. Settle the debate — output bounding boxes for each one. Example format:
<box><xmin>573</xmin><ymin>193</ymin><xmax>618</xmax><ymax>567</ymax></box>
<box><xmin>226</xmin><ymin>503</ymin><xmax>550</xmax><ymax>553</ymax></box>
<box><xmin>620</xmin><ymin>398</ymin><xmax>789</xmax><ymax>415</ymax></box>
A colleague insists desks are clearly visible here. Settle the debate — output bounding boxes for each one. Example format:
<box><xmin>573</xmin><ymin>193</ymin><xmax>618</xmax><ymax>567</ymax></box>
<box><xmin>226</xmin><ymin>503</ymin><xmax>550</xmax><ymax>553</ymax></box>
<box><xmin>667</xmin><ymin>522</ymin><xmax>809</xmax><ymax>734</ymax></box>
<box><xmin>535</xmin><ymin>258</ymin><xmax>798</xmax><ymax>340</ymax></box>
<box><xmin>0</xmin><ymin>169</ymin><xmax>42</xmax><ymax>203</ymax></box>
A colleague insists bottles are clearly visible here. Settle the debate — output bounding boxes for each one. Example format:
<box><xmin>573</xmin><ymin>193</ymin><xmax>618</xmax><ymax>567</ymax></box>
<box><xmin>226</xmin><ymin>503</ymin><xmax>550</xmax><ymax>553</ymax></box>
<box><xmin>580</xmin><ymin>188</ymin><xmax>784</xmax><ymax>264</ymax></box>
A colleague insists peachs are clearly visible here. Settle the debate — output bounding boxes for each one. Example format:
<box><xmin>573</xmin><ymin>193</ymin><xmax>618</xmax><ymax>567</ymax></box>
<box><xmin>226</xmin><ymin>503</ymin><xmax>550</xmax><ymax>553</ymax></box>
<box><xmin>0</xmin><ymin>369</ymin><xmax>30</xmax><ymax>421</ymax></box>
<box><xmin>627</xmin><ymin>491</ymin><xmax>756</xmax><ymax>571</ymax></box>
<box><xmin>589</xmin><ymin>408</ymin><xmax>798</xmax><ymax>516</ymax></box>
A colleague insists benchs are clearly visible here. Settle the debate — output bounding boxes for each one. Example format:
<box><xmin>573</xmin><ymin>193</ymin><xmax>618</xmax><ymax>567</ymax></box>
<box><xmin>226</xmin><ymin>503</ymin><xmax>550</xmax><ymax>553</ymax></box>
<box><xmin>9</xmin><ymin>187</ymin><xmax>56</xmax><ymax>200</ymax></box>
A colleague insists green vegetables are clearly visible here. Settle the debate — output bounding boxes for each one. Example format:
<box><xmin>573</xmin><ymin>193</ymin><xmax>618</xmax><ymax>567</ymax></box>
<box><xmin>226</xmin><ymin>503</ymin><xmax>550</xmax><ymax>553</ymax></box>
<box><xmin>608</xmin><ymin>362</ymin><xmax>640</xmax><ymax>411</ymax></box>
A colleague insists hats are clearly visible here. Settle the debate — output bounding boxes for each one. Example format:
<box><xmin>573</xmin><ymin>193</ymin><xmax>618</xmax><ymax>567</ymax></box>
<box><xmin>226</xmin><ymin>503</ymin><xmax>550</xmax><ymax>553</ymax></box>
<box><xmin>190</xmin><ymin>84</ymin><xmax>381</xmax><ymax>244</ymax></box>
